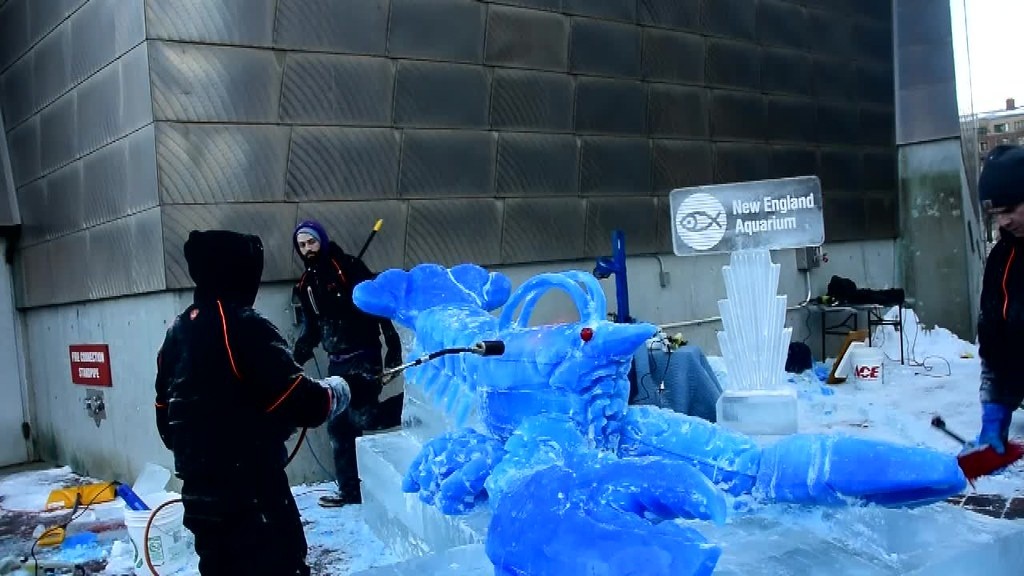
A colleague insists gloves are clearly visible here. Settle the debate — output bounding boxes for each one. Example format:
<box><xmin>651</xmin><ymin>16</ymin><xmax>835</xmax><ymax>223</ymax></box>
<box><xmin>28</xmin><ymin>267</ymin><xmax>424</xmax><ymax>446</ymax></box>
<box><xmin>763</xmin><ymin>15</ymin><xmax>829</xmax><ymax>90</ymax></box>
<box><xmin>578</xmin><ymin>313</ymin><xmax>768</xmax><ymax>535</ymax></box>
<box><xmin>346</xmin><ymin>372</ymin><xmax>382</xmax><ymax>409</ymax></box>
<box><xmin>980</xmin><ymin>403</ymin><xmax>1012</xmax><ymax>475</ymax></box>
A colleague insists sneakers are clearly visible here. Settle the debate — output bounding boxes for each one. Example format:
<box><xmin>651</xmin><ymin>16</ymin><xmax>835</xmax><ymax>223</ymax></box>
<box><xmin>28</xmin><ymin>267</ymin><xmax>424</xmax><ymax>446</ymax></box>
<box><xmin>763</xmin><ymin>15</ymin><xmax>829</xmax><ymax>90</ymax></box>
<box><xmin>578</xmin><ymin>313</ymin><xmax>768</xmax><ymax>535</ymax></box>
<box><xmin>319</xmin><ymin>494</ymin><xmax>361</xmax><ymax>507</ymax></box>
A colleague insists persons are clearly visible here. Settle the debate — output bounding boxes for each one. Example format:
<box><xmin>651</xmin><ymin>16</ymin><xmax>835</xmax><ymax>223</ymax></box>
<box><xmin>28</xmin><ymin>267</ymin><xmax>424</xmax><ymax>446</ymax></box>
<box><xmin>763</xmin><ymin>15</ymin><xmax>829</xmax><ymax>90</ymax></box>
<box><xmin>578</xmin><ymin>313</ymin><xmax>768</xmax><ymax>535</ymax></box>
<box><xmin>978</xmin><ymin>146</ymin><xmax>1024</xmax><ymax>473</ymax></box>
<box><xmin>156</xmin><ymin>231</ymin><xmax>384</xmax><ymax>576</ymax></box>
<box><xmin>292</xmin><ymin>220</ymin><xmax>404</xmax><ymax>507</ymax></box>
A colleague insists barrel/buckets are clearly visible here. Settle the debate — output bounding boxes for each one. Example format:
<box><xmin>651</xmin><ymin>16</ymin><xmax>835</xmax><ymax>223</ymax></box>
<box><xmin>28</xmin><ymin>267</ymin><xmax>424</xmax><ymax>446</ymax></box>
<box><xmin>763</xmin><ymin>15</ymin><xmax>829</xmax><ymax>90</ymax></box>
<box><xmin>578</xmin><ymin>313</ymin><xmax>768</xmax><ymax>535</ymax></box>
<box><xmin>851</xmin><ymin>347</ymin><xmax>883</xmax><ymax>392</ymax></box>
<box><xmin>123</xmin><ymin>493</ymin><xmax>191</xmax><ymax>576</ymax></box>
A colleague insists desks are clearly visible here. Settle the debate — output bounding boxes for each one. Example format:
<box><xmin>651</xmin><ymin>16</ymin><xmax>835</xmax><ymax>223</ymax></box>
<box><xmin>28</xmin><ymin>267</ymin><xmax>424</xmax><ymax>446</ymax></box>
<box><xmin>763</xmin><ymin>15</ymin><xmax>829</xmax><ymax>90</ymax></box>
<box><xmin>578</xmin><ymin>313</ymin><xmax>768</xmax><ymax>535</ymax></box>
<box><xmin>810</xmin><ymin>298</ymin><xmax>915</xmax><ymax>365</ymax></box>
<box><xmin>651</xmin><ymin>344</ymin><xmax>723</xmax><ymax>424</ymax></box>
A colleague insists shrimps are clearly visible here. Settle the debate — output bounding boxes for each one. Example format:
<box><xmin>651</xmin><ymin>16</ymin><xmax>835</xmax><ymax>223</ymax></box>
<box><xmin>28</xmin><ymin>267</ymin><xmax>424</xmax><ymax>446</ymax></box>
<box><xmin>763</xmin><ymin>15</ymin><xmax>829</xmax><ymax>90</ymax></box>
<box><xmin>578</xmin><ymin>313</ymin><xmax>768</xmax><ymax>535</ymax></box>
<box><xmin>352</xmin><ymin>263</ymin><xmax>969</xmax><ymax>576</ymax></box>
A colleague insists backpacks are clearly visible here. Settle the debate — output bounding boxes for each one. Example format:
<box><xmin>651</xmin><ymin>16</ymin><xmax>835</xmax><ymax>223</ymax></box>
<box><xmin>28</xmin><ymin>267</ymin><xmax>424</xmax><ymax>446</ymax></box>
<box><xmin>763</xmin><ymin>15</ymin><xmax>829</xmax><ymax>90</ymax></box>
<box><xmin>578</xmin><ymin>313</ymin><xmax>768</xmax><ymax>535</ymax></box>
<box><xmin>168</xmin><ymin>310</ymin><xmax>257</xmax><ymax>448</ymax></box>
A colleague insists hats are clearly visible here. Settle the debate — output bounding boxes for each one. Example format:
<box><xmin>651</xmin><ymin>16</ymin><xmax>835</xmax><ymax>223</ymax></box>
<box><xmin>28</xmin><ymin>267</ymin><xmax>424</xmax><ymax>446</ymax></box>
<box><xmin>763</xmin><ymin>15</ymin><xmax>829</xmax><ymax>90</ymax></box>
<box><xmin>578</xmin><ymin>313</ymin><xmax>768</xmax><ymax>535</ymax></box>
<box><xmin>978</xmin><ymin>144</ymin><xmax>1024</xmax><ymax>214</ymax></box>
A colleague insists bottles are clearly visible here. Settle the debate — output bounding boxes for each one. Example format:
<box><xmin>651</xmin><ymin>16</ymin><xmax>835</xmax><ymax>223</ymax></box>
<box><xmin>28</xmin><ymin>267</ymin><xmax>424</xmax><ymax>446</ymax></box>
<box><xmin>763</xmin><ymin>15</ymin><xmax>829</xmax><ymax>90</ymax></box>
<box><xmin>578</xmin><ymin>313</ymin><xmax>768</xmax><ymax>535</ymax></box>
<box><xmin>113</xmin><ymin>479</ymin><xmax>152</xmax><ymax>512</ymax></box>
